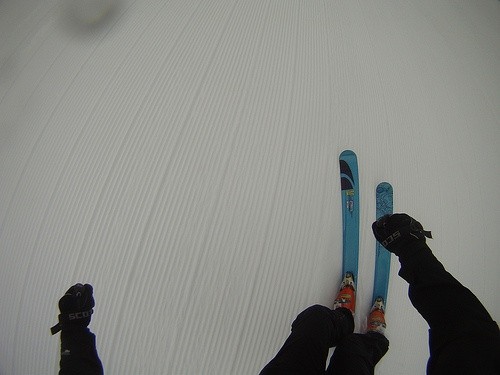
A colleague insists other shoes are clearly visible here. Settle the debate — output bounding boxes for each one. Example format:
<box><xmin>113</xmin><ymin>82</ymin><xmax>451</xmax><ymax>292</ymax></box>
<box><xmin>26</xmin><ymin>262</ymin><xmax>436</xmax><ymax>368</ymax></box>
<box><xmin>338</xmin><ymin>283</ymin><xmax>355</xmax><ymax>317</ymax></box>
<box><xmin>367</xmin><ymin>309</ymin><xmax>386</xmax><ymax>333</ymax></box>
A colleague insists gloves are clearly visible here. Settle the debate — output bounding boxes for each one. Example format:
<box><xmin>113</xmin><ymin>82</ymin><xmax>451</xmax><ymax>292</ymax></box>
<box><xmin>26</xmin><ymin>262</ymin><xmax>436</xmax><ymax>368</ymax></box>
<box><xmin>50</xmin><ymin>282</ymin><xmax>95</xmax><ymax>335</ymax></box>
<box><xmin>371</xmin><ymin>213</ymin><xmax>433</xmax><ymax>263</ymax></box>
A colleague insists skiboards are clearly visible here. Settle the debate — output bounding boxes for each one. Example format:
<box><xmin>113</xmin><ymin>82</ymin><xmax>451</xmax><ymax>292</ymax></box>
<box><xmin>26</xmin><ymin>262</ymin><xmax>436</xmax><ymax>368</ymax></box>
<box><xmin>339</xmin><ymin>149</ymin><xmax>394</xmax><ymax>311</ymax></box>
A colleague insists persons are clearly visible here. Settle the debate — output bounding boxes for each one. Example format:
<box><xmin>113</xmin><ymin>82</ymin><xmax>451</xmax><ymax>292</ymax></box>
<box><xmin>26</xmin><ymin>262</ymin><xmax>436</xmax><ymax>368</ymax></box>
<box><xmin>47</xmin><ymin>212</ymin><xmax>500</xmax><ymax>375</ymax></box>
